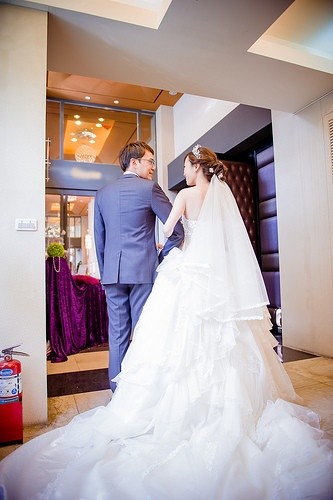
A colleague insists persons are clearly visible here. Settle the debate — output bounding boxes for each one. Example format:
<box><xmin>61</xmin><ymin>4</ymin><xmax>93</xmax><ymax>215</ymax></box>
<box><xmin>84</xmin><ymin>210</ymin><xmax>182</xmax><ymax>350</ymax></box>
<box><xmin>162</xmin><ymin>142</ymin><xmax>270</xmax><ymax>401</ymax></box>
<box><xmin>92</xmin><ymin>141</ymin><xmax>185</xmax><ymax>400</ymax></box>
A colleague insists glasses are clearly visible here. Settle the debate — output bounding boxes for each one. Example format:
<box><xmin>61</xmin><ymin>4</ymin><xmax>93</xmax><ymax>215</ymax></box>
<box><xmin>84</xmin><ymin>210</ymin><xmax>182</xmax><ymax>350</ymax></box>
<box><xmin>135</xmin><ymin>157</ymin><xmax>154</xmax><ymax>166</ymax></box>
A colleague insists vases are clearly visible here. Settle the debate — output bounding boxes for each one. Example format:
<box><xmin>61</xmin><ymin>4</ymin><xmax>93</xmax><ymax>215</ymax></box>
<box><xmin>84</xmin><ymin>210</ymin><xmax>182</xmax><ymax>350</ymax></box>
<box><xmin>47</xmin><ymin>242</ymin><xmax>64</xmax><ymax>257</ymax></box>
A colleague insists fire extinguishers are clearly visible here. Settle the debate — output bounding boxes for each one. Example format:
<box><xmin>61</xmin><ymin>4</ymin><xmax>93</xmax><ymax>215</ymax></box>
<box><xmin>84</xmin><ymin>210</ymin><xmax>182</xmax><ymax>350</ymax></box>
<box><xmin>0</xmin><ymin>344</ymin><xmax>30</xmax><ymax>446</ymax></box>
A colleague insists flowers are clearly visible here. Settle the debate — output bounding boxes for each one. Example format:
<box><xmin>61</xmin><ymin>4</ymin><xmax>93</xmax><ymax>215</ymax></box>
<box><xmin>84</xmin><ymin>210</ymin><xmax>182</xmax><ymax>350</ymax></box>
<box><xmin>209</xmin><ymin>168</ymin><xmax>213</xmax><ymax>174</ymax></box>
<box><xmin>218</xmin><ymin>172</ymin><xmax>223</xmax><ymax>180</ymax></box>
<box><xmin>44</xmin><ymin>224</ymin><xmax>67</xmax><ymax>245</ymax></box>
<box><xmin>192</xmin><ymin>144</ymin><xmax>202</xmax><ymax>159</ymax></box>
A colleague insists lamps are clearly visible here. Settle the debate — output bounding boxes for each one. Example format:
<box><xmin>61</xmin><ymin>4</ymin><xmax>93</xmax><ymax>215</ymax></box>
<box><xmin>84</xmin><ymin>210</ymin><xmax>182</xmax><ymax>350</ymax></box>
<box><xmin>75</xmin><ymin>145</ymin><xmax>97</xmax><ymax>163</ymax></box>
